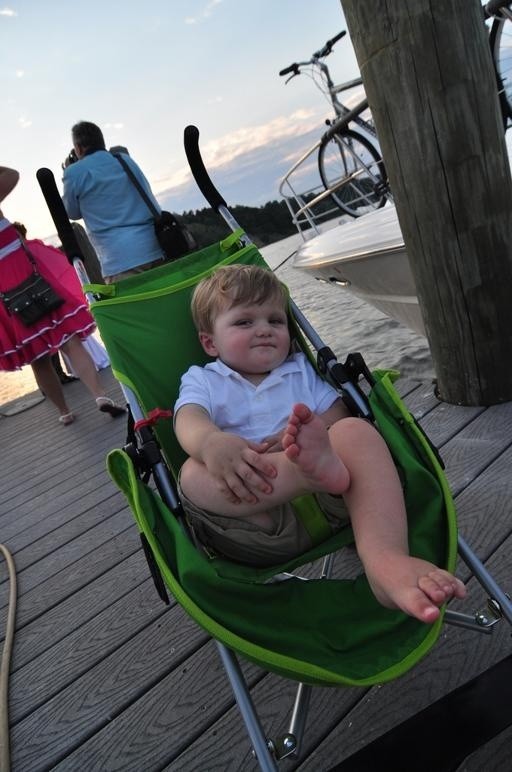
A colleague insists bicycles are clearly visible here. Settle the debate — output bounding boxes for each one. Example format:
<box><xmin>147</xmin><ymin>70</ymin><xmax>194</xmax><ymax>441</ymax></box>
<box><xmin>275</xmin><ymin>1</ymin><xmax>511</xmax><ymax>221</ymax></box>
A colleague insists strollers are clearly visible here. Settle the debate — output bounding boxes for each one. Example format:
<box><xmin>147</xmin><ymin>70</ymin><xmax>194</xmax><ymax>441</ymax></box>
<box><xmin>36</xmin><ymin>122</ymin><xmax>512</xmax><ymax>771</ymax></box>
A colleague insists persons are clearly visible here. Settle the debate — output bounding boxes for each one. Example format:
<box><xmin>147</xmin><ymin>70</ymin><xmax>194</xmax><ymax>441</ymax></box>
<box><xmin>61</xmin><ymin>121</ymin><xmax>169</xmax><ymax>285</ymax></box>
<box><xmin>0</xmin><ymin>166</ymin><xmax>128</xmax><ymax>426</ymax></box>
<box><xmin>172</xmin><ymin>262</ymin><xmax>468</xmax><ymax>624</ymax></box>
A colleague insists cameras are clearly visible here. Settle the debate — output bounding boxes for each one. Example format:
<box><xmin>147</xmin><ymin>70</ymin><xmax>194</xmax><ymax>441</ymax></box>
<box><xmin>62</xmin><ymin>151</ymin><xmax>77</xmax><ymax>169</ymax></box>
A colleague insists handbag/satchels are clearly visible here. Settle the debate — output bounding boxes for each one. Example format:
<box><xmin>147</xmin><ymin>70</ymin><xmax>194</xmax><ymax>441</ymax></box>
<box><xmin>2</xmin><ymin>269</ymin><xmax>64</xmax><ymax>329</ymax></box>
<box><xmin>154</xmin><ymin>211</ymin><xmax>195</xmax><ymax>260</ymax></box>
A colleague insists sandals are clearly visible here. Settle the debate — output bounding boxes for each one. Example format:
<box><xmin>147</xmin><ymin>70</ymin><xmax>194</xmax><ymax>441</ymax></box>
<box><xmin>96</xmin><ymin>397</ymin><xmax>125</xmax><ymax>416</ymax></box>
<box><xmin>58</xmin><ymin>412</ymin><xmax>76</xmax><ymax>426</ymax></box>
